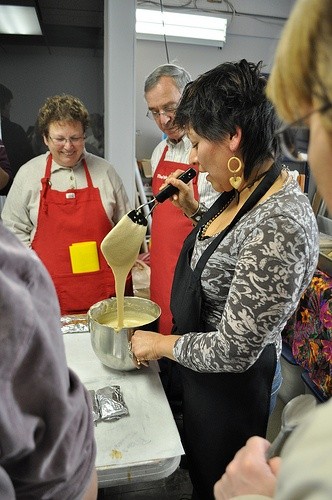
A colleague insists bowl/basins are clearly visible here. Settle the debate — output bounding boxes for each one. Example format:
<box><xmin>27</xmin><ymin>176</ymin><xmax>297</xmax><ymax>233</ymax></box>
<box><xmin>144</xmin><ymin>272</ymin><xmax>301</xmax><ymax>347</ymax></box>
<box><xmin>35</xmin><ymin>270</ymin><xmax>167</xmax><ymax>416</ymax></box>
<box><xmin>89</xmin><ymin>296</ymin><xmax>161</xmax><ymax>371</ymax></box>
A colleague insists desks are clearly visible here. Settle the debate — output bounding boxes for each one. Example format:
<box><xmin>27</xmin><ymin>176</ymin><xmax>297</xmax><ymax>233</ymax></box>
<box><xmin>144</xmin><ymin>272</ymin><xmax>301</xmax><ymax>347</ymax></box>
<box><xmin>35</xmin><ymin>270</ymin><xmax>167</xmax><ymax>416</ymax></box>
<box><xmin>59</xmin><ymin>313</ymin><xmax>184</xmax><ymax>489</ymax></box>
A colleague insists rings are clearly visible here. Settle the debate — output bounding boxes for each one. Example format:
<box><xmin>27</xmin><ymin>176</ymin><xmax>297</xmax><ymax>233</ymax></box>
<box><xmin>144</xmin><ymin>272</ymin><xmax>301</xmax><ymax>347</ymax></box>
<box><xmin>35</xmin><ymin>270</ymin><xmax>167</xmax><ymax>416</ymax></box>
<box><xmin>128</xmin><ymin>352</ymin><xmax>134</xmax><ymax>359</ymax></box>
<box><xmin>127</xmin><ymin>339</ymin><xmax>133</xmax><ymax>351</ymax></box>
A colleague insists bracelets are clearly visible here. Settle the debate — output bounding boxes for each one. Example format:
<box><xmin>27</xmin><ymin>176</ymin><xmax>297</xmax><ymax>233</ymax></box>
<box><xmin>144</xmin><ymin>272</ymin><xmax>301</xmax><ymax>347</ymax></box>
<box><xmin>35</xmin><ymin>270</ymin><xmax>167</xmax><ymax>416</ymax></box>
<box><xmin>183</xmin><ymin>201</ymin><xmax>207</xmax><ymax>224</ymax></box>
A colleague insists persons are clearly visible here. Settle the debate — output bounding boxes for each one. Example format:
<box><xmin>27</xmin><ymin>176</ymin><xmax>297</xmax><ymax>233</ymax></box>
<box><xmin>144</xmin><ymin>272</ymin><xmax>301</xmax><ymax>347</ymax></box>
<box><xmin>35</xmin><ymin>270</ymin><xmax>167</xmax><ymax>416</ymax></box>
<box><xmin>1</xmin><ymin>214</ymin><xmax>98</xmax><ymax>500</ymax></box>
<box><xmin>83</xmin><ymin>112</ymin><xmax>105</xmax><ymax>158</ymax></box>
<box><xmin>141</xmin><ymin>62</ymin><xmax>223</xmax><ymax>335</ymax></box>
<box><xmin>1</xmin><ymin>84</ymin><xmax>29</xmax><ymax>165</ymax></box>
<box><xmin>0</xmin><ymin>93</ymin><xmax>135</xmax><ymax>314</ymax></box>
<box><xmin>0</xmin><ymin>138</ymin><xmax>13</xmax><ymax>190</ymax></box>
<box><xmin>214</xmin><ymin>0</ymin><xmax>332</xmax><ymax>500</ymax></box>
<box><xmin>99</xmin><ymin>58</ymin><xmax>318</xmax><ymax>499</ymax></box>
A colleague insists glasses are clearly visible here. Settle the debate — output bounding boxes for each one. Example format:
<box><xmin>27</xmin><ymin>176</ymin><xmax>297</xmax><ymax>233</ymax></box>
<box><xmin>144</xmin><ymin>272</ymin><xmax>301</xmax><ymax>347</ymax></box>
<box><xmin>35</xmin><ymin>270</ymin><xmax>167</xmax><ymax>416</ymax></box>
<box><xmin>49</xmin><ymin>130</ymin><xmax>86</xmax><ymax>145</ymax></box>
<box><xmin>146</xmin><ymin>97</ymin><xmax>181</xmax><ymax>120</ymax></box>
<box><xmin>275</xmin><ymin>104</ymin><xmax>332</xmax><ymax>162</ymax></box>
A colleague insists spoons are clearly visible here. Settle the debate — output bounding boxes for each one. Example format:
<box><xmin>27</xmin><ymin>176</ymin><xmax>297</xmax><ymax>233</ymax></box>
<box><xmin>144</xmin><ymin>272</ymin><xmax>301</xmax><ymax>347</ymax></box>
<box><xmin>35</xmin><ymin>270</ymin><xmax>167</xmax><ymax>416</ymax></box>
<box><xmin>266</xmin><ymin>394</ymin><xmax>317</xmax><ymax>461</ymax></box>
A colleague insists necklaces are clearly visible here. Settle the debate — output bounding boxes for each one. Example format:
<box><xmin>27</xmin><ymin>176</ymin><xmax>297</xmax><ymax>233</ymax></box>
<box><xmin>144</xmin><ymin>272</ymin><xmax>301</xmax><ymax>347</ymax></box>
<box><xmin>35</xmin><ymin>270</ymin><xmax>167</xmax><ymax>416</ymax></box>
<box><xmin>201</xmin><ymin>186</ymin><xmax>241</xmax><ymax>238</ymax></box>
